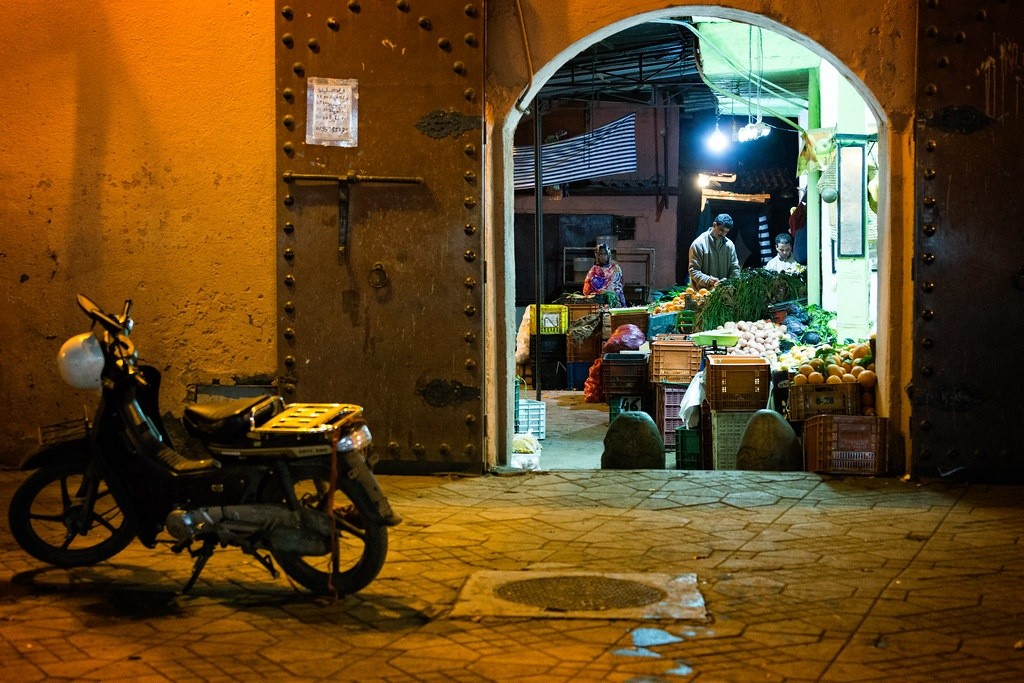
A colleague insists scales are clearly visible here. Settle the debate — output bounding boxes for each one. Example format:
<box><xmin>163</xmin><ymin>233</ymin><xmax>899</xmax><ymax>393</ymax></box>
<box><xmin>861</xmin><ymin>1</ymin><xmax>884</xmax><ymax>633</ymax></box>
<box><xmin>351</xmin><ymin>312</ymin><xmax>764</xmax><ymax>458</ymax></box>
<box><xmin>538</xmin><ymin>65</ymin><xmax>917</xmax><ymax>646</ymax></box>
<box><xmin>693</xmin><ymin>335</ymin><xmax>741</xmax><ymax>371</ymax></box>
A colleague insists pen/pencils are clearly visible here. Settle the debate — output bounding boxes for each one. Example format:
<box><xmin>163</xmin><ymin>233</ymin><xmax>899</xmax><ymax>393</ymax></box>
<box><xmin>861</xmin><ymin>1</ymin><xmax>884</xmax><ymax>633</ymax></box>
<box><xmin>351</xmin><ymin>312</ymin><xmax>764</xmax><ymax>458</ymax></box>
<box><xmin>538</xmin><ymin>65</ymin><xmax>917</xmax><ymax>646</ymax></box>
<box><xmin>710</xmin><ymin>275</ymin><xmax>713</xmax><ymax>277</ymax></box>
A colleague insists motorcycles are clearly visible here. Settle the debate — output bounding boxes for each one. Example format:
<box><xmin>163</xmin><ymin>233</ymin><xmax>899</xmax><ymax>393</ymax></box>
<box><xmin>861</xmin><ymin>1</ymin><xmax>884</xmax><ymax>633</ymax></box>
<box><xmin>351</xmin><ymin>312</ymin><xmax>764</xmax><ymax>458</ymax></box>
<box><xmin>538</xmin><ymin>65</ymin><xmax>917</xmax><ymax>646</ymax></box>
<box><xmin>7</xmin><ymin>294</ymin><xmax>403</xmax><ymax>601</ymax></box>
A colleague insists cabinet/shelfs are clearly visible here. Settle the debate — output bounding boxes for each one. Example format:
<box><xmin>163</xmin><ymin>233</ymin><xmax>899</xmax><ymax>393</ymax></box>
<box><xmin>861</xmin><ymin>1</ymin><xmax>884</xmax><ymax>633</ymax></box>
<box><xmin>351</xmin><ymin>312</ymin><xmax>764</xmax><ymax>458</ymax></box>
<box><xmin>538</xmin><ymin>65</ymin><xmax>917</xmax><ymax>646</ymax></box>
<box><xmin>562</xmin><ymin>247</ymin><xmax>655</xmax><ymax>306</ymax></box>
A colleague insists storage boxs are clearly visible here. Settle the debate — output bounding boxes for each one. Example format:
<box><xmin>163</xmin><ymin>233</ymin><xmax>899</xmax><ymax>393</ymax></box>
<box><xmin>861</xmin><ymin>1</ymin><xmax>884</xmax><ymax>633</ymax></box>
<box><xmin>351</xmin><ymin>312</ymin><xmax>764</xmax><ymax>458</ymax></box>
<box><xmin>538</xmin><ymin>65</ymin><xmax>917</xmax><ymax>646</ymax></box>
<box><xmin>802</xmin><ymin>414</ymin><xmax>890</xmax><ymax>476</ymax></box>
<box><xmin>567</xmin><ymin>335</ymin><xmax>602</xmax><ymax>360</ymax></box>
<box><xmin>649</xmin><ymin>341</ymin><xmax>703</xmax><ymax>382</ymax></box>
<box><xmin>567</xmin><ymin>304</ymin><xmax>601</xmax><ymax>332</ymax></box>
<box><xmin>674</xmin><ymin>425</ymin><xmax>700</xmax><ymax>470</ymax></box>
<box><xmin>607</xmin><ymin>394</ymin><xmax>643</xmax><ymax>425</ymax></box>
<box><xmin>656</xmin><ymin>384</ymin><xmax>688</xmax><ymax>446</ymax></box>
<box><xmin>513</xmin><ymin>378</ymin><xmax>546</xmax><ymax>440</ymax></box>
<box><xmin>566</xmin><ymin>361</ymin><xmax>593</xmax><ymax>390</ymax></box>
<box><xmin>530</xmin><ymin>304</ymin><xmax>567</xmax><ymax>333</ymax></box>
<box><xmin>602</xmin><ymin>353</ymin><xmax>647</xmax><ymax>395</ymax></box>
<box><xmin>710</xmin><ymin>410</ymin><xmax>754</xmax><ymax>471</ymax></box>
<box><xmin>529</xmin><ymin>360</ymin><xmax>568</xmax><ymax>390</ymax></box>
<box><xmin>706</xmin><ymin>354</ymin><xmax>771</xmax><ymax>410</ymax></box>
<box><xmin>608</xmin><ymin>306</ymin><xmax>650</xmax><ymax>339</ymax></box>
<box><xmin>790</xmin><ymin>382</ymin><xmax>862</xmax><ymax>420</ymax></box>
<box><xmin>528</xmin><ymin>332</ymin><xmax>567</xmax><ymax>362</ymax></box>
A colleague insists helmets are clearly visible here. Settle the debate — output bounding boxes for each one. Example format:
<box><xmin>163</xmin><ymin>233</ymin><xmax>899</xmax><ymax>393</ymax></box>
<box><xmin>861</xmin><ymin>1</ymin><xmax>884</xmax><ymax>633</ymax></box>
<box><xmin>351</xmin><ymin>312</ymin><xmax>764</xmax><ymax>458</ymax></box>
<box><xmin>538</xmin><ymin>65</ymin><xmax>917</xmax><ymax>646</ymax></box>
<box><xmin>57</xmin><ymin>332</ymin><xmax>105</xmax><ymax>390</ymax></box>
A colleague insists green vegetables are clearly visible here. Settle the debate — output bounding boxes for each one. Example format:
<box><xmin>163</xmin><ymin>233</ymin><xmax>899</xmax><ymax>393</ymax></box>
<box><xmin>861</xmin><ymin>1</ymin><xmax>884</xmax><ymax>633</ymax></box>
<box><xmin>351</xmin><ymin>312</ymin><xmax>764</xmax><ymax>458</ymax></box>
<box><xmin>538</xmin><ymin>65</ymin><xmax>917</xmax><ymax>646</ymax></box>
<box><xmin>693</xmin><ymin>269</ymin><xmax>806</xmax><ymax>331</ymax></box>
<box><xmin>562</xmin><ymin>290</ymin><xmax>622</xmax><ymax>308</ymax></box>
<box><xmin>797</xmin><ymin>302</ymin><xmax>839</xmax><ymax>343</ymax></box>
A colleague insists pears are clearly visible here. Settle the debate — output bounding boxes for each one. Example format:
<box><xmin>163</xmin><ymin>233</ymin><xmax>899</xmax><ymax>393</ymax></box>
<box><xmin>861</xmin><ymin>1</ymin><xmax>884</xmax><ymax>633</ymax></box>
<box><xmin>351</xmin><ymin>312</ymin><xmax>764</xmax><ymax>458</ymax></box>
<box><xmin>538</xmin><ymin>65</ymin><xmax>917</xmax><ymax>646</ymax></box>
<box><xmin>779</xmin><ymin>344</ymin><xmax>831</xmax><ymax>371</ymax></box>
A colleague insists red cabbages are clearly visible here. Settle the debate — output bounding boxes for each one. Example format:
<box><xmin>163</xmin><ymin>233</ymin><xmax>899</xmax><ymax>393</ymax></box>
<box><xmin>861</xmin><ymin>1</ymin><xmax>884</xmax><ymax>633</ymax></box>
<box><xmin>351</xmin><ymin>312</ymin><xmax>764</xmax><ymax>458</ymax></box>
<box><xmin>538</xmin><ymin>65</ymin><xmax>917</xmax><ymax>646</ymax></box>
<box><xmin>784</xmin><ymin>304</ymin><xmax>820</xmax><ymax>345</ymax></box>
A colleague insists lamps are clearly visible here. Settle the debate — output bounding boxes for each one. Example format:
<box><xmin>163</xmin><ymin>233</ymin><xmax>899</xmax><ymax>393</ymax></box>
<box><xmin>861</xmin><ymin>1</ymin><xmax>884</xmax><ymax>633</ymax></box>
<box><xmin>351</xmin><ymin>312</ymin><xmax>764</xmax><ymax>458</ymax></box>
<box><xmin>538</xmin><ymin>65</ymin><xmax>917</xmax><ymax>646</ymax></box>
<box><xmin>732</xmin><ymin>26</ymin><xmax>772</xmax><ymax>142</ymax></box>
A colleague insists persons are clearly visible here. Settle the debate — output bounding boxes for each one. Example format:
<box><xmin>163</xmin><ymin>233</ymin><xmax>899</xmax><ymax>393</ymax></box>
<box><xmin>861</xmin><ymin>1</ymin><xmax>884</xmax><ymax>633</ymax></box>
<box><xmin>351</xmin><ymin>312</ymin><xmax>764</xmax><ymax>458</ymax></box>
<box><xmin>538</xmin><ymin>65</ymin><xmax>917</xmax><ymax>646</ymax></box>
<box><xmin>688</xmin><ymin>214</ymin><xmax>741</xmax><ymax>292</ymax></box>
<box><xmin>583</xmin><ymin>242</ymin><xmax>626</xmax><ymax>309</ymax></box>
<box><xmin>765</xmin><ymin>234</ymin><xmax>802</xmax><ymax>276</ymax></box>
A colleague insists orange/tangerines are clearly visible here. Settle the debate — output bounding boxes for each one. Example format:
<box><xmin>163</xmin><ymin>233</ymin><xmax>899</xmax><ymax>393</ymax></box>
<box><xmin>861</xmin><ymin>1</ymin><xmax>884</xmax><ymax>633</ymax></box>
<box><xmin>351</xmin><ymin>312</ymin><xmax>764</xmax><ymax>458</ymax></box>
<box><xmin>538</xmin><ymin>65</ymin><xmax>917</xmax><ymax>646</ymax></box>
<box><xmin>654</xmin><ymin>286</ymin><xmax>712</xmax><ymax>314</ymax></box>
<box><xmin>794</xmin><ymin>333</ymin><xmax>877</xmax><ymax>414</ymax></box>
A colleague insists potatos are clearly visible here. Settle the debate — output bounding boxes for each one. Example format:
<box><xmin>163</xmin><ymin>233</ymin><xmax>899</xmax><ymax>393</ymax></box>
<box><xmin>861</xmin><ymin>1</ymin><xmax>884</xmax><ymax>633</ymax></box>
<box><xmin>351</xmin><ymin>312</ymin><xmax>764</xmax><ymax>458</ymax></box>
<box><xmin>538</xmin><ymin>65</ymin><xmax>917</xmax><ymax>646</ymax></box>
<box><xmin>717</xmin><ymin>319</ymin><xmax>791</xmax><ymax>357</ymax></box>
<box><xmin>584</xmin><ymin>366</ymin><xmax>603</xmax><ymax>403</ymax></box>
